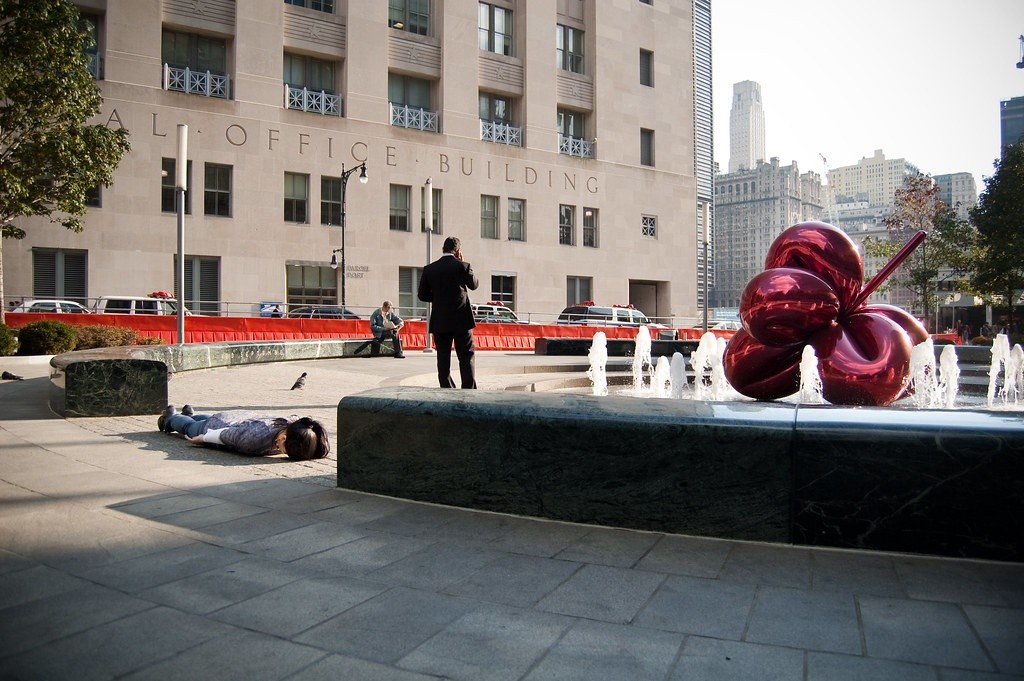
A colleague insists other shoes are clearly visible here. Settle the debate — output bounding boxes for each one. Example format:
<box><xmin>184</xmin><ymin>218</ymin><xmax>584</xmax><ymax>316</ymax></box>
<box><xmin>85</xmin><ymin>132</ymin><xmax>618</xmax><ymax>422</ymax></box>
<box><xmin>394</xmin><ymin>352</ymin><xmax>406</xmax><ymax>358</ymax></box>
<box><xmin>181</xmin><ymin>404</ymin><xmax>194</xmax><ymax>417</ymax></box>
<box><xmin>158</xmin><ymin>404</ymin><xmax>174</xmax><ymax>432</ymax></box>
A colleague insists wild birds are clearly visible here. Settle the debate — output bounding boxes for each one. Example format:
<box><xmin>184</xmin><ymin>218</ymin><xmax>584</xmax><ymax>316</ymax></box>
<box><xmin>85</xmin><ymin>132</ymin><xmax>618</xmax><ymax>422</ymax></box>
<box><xmin>290</xmin><ymin>372</ymin><xmax>307</xmax><ymax>390</ymax></box>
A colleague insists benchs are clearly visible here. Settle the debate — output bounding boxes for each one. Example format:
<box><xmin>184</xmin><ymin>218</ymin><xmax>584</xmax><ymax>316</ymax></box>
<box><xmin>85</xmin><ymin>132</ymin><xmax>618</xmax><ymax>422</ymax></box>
<box><xmin>336</xmin><ymin>384</ymin><xmax>1024</xmax><ymax>566</ymax></box>
<box><xmin>934</xmin><ymin>344</ymin><xmax>1024</xmax><ymax>366</ymax></box>
<box><xmin>47</xmin><ymin>338</ymin><xmax>402</xmax><ymax>420</ymax></box>
<box><xmin>535</xmin><ymin>336</ymin><xmax>701</xmax><ymax>356</ymax></box>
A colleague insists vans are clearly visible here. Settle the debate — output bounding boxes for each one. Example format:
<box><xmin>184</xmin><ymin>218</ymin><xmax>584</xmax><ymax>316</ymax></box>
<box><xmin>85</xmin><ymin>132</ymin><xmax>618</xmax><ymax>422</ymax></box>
<box><xmin>92</xmin><ymin>291</ymin><xmax>207</xmax><ymax>317</ymax></box>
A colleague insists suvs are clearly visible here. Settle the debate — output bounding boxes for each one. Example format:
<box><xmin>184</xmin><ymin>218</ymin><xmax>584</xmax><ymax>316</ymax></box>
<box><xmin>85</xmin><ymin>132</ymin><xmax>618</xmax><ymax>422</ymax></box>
<box><xmin>557</xmin><ymin>300</ymin><xmax>667</xmax><ymax>328</ymax></box>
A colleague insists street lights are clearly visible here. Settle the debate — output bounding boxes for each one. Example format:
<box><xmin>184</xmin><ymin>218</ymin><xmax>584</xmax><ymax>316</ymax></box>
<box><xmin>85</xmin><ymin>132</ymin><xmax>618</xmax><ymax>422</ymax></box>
<box><xmin>330</xmin><ymin>162</ymin><xmax>368</xmax><ymax>306</ymax></box>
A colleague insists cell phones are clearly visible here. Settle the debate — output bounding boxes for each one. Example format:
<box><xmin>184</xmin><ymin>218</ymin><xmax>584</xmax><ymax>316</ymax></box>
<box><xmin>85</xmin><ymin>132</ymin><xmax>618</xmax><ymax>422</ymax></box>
<box><xmin>457</xmin><ymin>248</ymin><xmax>461</xmax><ymax>259</ymax></box>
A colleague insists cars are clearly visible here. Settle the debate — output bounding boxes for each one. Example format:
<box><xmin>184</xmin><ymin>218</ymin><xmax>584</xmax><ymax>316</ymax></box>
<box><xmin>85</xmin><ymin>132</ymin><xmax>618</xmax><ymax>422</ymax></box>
<box><xmin>471</xmin><ymin>302</ymin><xmax>528</xmax><ymax>324</ymax></box>
<box><xmin>280</xmin><ymin>305</ymin><xmax>361</xmax><ymax>319</ymax></box>
<box><xmin>12</xmin><ymin>300</ymin><xmax>91</xmax><ymax>314</ymax></box>
<box><xmin>693</xmin><ymin>322</ymin><xmax>742</xmax><ymax>330</ymax></box>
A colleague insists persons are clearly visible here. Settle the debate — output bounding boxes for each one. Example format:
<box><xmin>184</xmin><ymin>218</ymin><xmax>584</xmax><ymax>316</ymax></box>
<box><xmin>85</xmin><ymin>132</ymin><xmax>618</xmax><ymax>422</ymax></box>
<box><xmin>370</xmin><ymin>301</ymin><xmax>406</xmax><ymax>358</ymax></box>
<box><xmin>157</xmin><ymin>404</ymin><xmax>331</xmax><ymax>461</ymax></box>
<box><xmin>955</xmin><ymin>319</ymin><xmax>1009</xmax><ymax>345</ymax></box>
<box><xmin>271</xmin><ymin>305</ymin><xmax>282</xmax><ymax>318</ymax></box>
<box><xmin>417</xmin><ymin>236</ymin><xmax>479</xmax><ymax>389</ymax></box>
